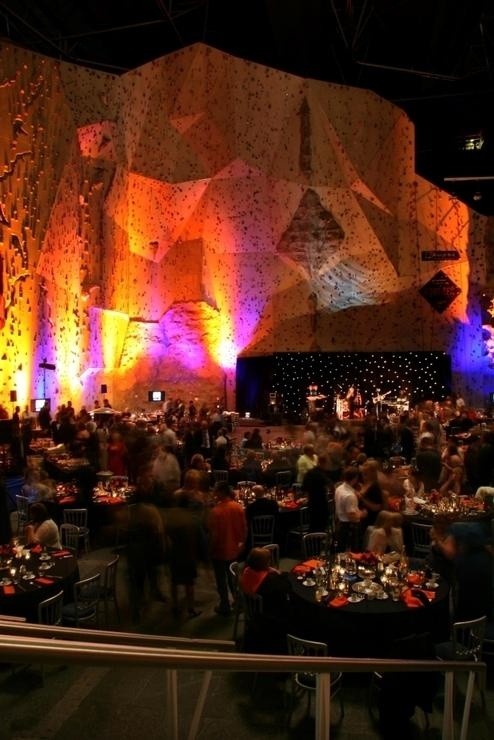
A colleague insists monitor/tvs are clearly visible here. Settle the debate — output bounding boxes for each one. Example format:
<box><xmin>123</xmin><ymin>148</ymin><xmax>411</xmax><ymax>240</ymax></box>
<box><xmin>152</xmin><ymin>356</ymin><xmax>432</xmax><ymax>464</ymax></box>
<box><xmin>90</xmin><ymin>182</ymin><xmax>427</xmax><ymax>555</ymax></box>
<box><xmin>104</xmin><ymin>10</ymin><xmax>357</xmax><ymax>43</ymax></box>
<box><xmin>148</xmin><ymin>391</ymin><xmax>165</xmax><ymax>402</ymax></box>
<box><xmin>30</xmin><ymin>398</ymin><xmax>51</xmax><ymax>412</ymax></box>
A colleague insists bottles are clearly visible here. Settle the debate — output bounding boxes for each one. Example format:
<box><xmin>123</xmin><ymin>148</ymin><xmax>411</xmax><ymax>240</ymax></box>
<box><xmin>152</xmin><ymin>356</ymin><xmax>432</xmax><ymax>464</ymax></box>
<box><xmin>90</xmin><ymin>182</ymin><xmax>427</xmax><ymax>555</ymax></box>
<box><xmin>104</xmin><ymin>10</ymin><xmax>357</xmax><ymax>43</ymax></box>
<box><xmin>393</xmin><ymin>584</ymin><xmax>400</xmax><ymax>601</ymax></box>
<box><xmin>400</xmin><ymin>544</ymin><xmax>408</xmax><ymax>563</ymax></box>
<box><xmin>348</xmin><ymin>553</ymin><xmax>354</xmax><ymax>571</ymax></box>
<box><xmin>376</xmin><ymin>552</ymin><xmax>385</xmax><ymax>577</ymax></box>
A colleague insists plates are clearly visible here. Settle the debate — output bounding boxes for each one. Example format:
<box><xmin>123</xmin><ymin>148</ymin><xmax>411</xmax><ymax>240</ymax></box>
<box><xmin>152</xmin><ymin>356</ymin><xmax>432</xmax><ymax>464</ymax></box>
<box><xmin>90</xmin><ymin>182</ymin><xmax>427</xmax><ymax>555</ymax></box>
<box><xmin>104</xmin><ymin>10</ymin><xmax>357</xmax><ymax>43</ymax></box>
<box><xmin>351</xmin><ymin>581</ymin><xmax>383</xmax><ymax>595</ymax></box>
<box><xmin>426</xmin><ymin>583</ymin><xmax>439</xmax><ymax>588</ymax></box>
<box><xmin>0</xmin><ymin>581</ymin><xmax>12</xmax><ymax>586</ymax></box>
<box><xmin>22</xmin><ymin>575</ymin><xmax>36</xmax><ymax>580</ymax></box>
<box><xmin>38</xmin><ymin>556</ymin><xmax>51</xmax><ymax>561</ymax></box>
<box><xmin>314</xmin><ymin>590</ymin><xmax>328</xmax><ymax>596</ymax></box>
<box><xmin>347</xmin><ymin>597</ymin><xmax>361</xmax><ymax>603</ymax></box>
<box><xmin>39</xmin><ymin>566</ymin><xmax>51</xmax><ymax>570</ymax></box>
<box><xmin>303</xmin><ymin>580</ymin><xmax>316</xmax><ymax>587</ymax></box>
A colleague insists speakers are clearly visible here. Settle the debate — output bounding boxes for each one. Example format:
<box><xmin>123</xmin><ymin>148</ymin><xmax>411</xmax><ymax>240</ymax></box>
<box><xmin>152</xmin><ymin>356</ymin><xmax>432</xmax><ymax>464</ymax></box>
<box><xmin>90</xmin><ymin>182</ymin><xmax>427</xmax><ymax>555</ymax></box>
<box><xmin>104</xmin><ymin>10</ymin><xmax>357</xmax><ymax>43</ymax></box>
<box><xmin>10</xmin><ymin>390</ymin><xmax>17</xmax><ymax>402</ymax></box>
<box><xmin>101</xmin><ymin>384</ymin><xmax>107</xmax><ymax>393</ymax></box>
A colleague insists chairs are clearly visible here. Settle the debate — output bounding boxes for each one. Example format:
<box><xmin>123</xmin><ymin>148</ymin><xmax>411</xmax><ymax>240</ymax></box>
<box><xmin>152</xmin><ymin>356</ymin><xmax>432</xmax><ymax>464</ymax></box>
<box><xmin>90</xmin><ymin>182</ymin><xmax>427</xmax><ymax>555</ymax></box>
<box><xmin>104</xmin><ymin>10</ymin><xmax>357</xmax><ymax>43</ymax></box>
<box><xmin>0</xmin><ymin>431</ymin><xmax>494</xmax><ymax>728</ymax></box>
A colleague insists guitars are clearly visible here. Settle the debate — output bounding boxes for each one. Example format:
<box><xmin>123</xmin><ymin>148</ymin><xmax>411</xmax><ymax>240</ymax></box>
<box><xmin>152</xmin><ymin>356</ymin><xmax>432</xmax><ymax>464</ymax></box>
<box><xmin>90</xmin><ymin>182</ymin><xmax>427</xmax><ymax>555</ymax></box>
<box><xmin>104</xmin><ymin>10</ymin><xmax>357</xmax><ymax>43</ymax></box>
<box><xmin>372</xmin><ymin>391</ymin><xmax>391</xmax><ymax>404</ymax></box>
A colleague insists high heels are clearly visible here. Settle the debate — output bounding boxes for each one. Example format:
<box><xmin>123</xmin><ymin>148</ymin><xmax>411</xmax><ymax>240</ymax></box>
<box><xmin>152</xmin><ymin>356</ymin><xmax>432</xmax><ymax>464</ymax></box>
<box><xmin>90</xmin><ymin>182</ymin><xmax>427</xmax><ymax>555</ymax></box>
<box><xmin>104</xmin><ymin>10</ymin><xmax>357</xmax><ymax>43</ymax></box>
<box><xmin>188</xmin><ymin>606</ymin><xmax>203</xmax><ymax>617</ymax></box>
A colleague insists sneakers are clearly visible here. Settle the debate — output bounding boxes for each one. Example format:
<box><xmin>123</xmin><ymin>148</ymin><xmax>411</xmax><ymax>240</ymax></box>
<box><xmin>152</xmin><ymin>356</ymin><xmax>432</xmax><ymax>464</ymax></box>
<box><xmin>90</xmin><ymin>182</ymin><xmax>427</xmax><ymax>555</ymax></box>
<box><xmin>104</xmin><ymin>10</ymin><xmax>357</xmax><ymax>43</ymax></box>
<box><xmin>214</xmin><ymin>605</ymin><xmax>232</xmax><ymax>617</ymax></box>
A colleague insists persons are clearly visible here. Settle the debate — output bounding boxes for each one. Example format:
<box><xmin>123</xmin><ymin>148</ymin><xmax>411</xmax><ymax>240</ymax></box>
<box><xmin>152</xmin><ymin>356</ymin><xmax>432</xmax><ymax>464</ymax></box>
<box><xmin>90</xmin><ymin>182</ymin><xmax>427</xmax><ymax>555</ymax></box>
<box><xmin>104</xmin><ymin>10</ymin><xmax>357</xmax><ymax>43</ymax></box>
<box><xmin>299</xmin><ymin>454</ymin><xmax>342</xmax><ymax>534</ymax></box>
<box><xmin>440</xmin><ymin>526</ymin><xmax>491</xmax><ymax>647</ymax></box>
<box><xmin>117</xmin><ymin>487</ymin><xmax>166</xmax><ymax>624</ymax></box>
<box><xmin>238</xmin><ymin>547</ymin><xmax>282</xmax><ymax>617</ymax></box>
<box><xmin>207</xmin><ymin>480</ymin><xmax>246</xmax><ymax>616</ymax></box>
<box><xmin>335</xmin><ymin>467</ymin><xmax>368</xmax><ymax>554</ymax></box>
<box><xmin>21</xmin><ymin>467</ymin><xmax>57</xmax><ymax>501</ymax></box>
<box><xmin>1</xmin><ymin>387</ymin><xmax>493</xmax><ymax>497</ymax></box>
<box><xmin>24</xmin><ymin>503</ymin><xmax>63</xmax><ymax>552</ymax></box>
<box><xmin>166</xmin><ymin>494</ymin><xmax>207</xmax><ymax>617</ymax></box>
<box><xmin>174</xmin><ymin>469</ymin><xmax>205</xmax><ymax>504</ymax></box>
<box><xmin>366</xmin><ymin>510</ymin><xmax>404</xmax><ymax>554</ymax></box>
<box><xmin>360</xmin><ymin>459</ymin><xmax>383</xmax><ymax>551</ymax></box>
<box><xmin>413</xmin><ymin>521</ymin><xmax>451</xmax><ymax>580</ymax></box>
<box><xmin>246</xmin><ymin>485</ymin><xmax>280</xmax><ymax>535</ymax></box>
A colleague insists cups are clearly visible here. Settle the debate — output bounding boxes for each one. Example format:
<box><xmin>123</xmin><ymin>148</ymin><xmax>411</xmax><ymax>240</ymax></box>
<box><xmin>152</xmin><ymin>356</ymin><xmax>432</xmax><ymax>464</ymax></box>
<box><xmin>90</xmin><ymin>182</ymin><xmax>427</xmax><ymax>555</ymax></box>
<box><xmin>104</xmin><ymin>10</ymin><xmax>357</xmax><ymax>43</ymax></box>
<box><xmin>42</xmin><ymin>553</ymin><xmax>47</xmax><ymax>559</ymax></box>
<box><xmin>352</xmin><ymin>593</ymin><xmax>357</xmax><ymax>601</ymax></box>
<box><xmin>306</xmin><ymin>578</ymin><xmax>312</xmax><ymax>585</ymax></box>
<box><xmin>2</xmin><ymin>578</ymin><xmax>8</xmax><ymax>583</ymax></box>
<box><xmin>42</xmin><ymin>563</ymin><xmax>47</xmax><ymax>568</ymax></box>
<box><xmin>318</xmin><ymin>586</ymin><xmax>324</xmax><ymax>595</ymax></box>
<box><xmin>26</xmin><ymin>572</ymin><xmax>32</xmax><ymax>577</ymax></box>
